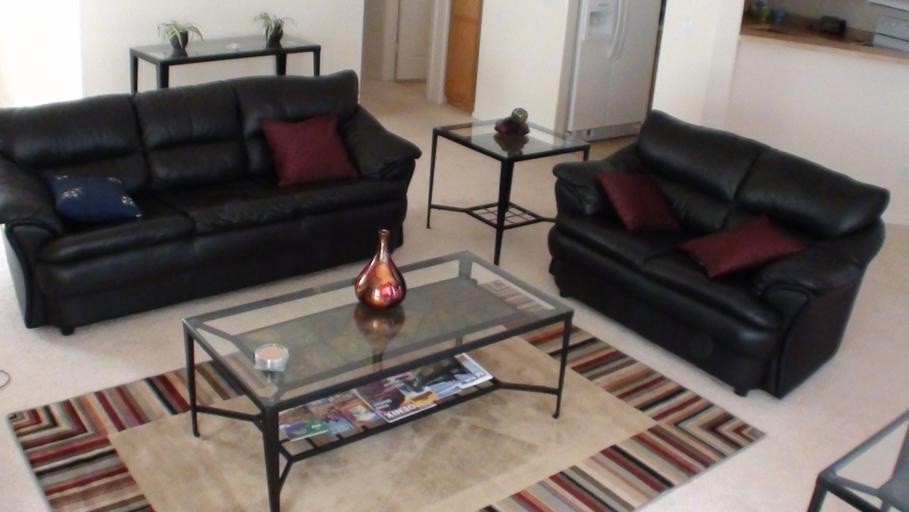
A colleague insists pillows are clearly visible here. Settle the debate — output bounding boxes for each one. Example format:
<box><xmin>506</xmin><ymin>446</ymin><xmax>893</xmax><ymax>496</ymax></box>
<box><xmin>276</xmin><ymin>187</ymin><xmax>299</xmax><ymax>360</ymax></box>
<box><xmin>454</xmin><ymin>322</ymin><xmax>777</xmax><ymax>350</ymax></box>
<box><xmin>593</xmin><ymin>171</ymin><xmax>807</xmax><ymax>282</ymax></box>
<box><xmin>40</xmin><ymin>163</ymin><xmax>141</xmax><ymax>222</ymax></box>
<box><xmin>259</xmin><ymin>112</ymin><xmax>359</xmax><ymax>186</ymax></box>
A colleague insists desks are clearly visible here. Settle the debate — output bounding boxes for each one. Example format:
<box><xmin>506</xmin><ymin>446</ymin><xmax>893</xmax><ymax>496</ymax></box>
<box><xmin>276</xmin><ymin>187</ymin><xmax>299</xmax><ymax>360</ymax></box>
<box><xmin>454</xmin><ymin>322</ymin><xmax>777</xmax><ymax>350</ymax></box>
<box><xmin>129</xmin><ymin>34</ymin><xmax>321</xmax><ymax>96</ymax></box>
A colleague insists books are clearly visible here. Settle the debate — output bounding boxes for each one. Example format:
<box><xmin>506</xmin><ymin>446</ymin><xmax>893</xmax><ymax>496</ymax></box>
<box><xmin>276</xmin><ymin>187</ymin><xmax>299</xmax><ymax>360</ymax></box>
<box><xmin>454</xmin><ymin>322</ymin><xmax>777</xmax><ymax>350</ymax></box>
<box><xmin>277</xmin><ymin>351</ymin><xmax>495</xmax><ymax>443</ymax></box>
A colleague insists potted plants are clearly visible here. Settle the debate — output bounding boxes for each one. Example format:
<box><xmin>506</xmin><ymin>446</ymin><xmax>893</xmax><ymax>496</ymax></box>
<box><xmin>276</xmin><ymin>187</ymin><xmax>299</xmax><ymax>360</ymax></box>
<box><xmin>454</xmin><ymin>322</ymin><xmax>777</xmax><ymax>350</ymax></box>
<box><xmin>253</xmin><ymin>10</ymin><xmax>298</xmax><ymax>45</ymax></box>
<box><xmin>155</xmin><ymin>19</ymin><xmax>203</xmax><ymax>50</ymax></box>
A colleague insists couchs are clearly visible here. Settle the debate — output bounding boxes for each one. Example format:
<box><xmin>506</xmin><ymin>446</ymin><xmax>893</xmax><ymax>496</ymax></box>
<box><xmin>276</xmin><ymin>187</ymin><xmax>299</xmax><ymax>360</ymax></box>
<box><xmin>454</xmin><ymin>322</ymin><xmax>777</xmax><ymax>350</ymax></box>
<box><xmin>548</xmin><ymin>110</ymin><xmax>893</xmax><ymax>400</ymax></box>
<box><xmin>0</xmin><ymin>70</ymin><xmax>422</xmax><ymax>336</ymax></box>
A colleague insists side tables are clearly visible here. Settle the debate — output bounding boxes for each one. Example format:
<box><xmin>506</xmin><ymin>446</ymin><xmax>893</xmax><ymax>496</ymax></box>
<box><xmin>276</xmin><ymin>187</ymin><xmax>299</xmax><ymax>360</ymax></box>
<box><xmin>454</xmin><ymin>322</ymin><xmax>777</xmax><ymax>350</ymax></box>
<box><xmin>426</xmin><ymin>118</ymin><xmax>591</xmax><ymax>265</ymax></box>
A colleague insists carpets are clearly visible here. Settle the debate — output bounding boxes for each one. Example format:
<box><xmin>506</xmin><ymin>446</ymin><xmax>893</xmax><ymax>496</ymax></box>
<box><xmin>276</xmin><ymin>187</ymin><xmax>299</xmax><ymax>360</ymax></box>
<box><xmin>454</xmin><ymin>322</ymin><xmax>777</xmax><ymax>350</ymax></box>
<box><xmin>7</xmin><ymin>279</ymin><xmax>767</xmax><ymax>512</ymax></box>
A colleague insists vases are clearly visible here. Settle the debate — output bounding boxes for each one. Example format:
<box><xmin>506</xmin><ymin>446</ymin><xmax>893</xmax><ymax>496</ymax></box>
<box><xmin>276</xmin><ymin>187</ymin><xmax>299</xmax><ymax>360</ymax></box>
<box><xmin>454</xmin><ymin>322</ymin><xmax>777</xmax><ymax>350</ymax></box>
<box><xmin>355</xmin><ymin>230</ymin><xmax>407</xmax><ymax>310</ymax></box>
